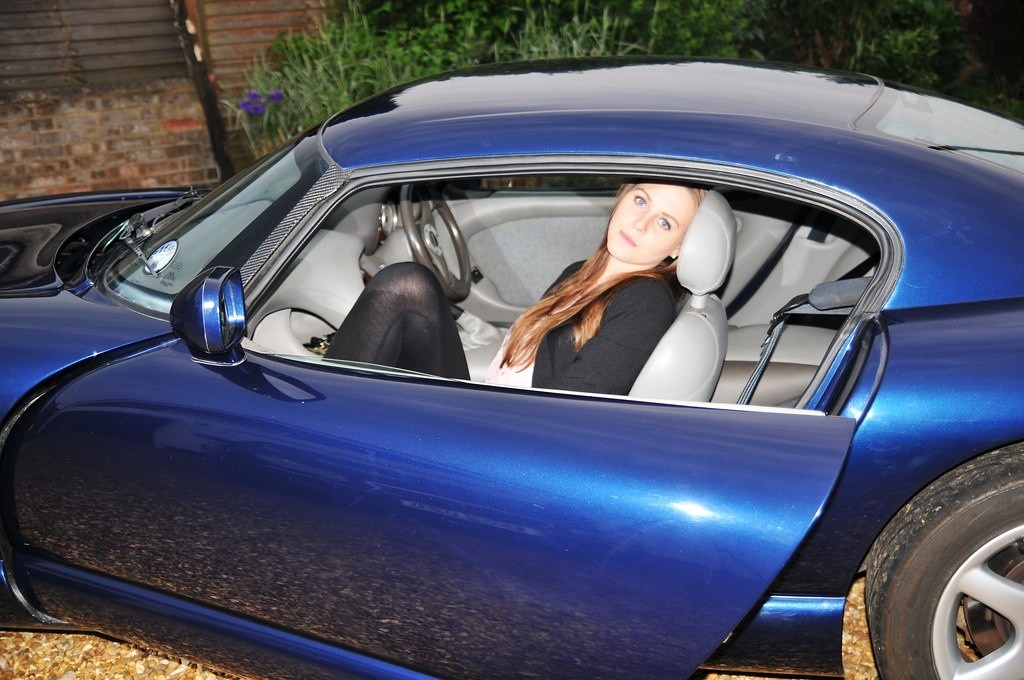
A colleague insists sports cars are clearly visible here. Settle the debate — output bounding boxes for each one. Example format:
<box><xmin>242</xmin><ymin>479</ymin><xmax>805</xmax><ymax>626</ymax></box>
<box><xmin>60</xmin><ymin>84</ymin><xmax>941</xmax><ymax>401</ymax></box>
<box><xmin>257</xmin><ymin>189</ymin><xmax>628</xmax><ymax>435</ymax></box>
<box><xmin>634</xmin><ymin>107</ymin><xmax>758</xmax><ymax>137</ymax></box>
<box><xmin>1</xmin><ymin>51</ymin><xmax>1024</xmax><ymax>680</ymax></box>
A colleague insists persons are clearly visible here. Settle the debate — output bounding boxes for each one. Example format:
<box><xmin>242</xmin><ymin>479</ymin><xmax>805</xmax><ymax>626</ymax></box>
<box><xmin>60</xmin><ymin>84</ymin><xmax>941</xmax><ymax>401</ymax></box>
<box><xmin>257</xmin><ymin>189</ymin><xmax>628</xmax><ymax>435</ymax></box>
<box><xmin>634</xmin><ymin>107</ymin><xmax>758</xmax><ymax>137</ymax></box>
<box><xmin>319</xmin><ymin>179</ymin><xmax>717</xmax><ymax>391</ymax></box>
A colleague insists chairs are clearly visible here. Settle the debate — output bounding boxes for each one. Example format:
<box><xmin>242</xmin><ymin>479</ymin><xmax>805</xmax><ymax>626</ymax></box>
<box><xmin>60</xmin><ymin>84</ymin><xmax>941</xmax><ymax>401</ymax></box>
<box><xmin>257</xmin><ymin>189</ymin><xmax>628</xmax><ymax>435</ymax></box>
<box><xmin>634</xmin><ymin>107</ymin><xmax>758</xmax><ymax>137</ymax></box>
<box><xmin>627</xmin><ymin>189</ymin><xmax>737</xmax><ymax>403</ymax></box>
<box><xmin>711</xmin><ymin>235</ymin><xmax>881</xmax><ymax>407</ymax></box>
